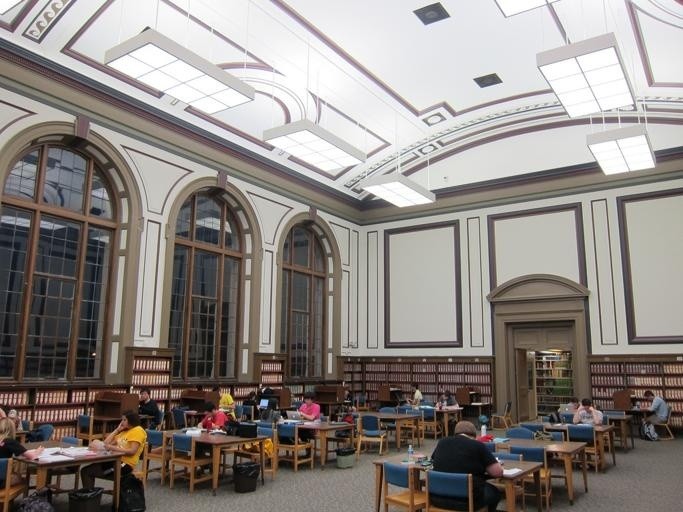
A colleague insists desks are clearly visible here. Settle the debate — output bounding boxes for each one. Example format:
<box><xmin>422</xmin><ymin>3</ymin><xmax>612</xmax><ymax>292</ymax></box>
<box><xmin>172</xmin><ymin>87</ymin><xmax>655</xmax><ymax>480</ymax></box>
<box><xmin>541</xmin><ymin>423</ymin><xmax>616</xmax><ymax>472</ymax></box>
<box><xmin>372</xmin><ymin>453</ymin><xmax>543</xmax><ymax>511</ymax></box>
<box><xmin>463</xmin><ymin>402</ymin><xmax>493</xmax><ymax>428</ymax></box>
<box><xmin>610</xmin><ymin>406</ymin><xmax>654</xmax><ymax>454</ymax></box>
<box><xmin>485</xmin><ymin>437</ymin><xmax>589</xmax><ymax>504</ymax></box>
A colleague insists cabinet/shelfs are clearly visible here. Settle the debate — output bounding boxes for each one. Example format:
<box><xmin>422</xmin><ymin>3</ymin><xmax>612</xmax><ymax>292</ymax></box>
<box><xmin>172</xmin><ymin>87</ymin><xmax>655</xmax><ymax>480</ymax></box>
<box><xmin>587</xmin><ymin>353</ymin><xmax>683</xmax><ymax>437</ymax></box>
<box><xmin>335</xmin><ymin>356</ymin><xmax>496</xmax><ymax>416</ymax></box>
<box><xmin>534</xmin><ymin>352</ymin><xmax>573</xmax><ymax>414</ymax></box>
<box><xmin>0</xmin><ymin>347</ymin><xmax>342</xmax><ymax>441</ymax></box>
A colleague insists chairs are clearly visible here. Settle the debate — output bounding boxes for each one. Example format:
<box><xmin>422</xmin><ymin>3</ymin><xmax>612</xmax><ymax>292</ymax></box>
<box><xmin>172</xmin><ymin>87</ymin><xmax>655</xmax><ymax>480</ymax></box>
<box><xmin>654</xmin><ymin>408</ymin><xmax>672</xmax><ymax>439</ymax></box>
<box><xmin>603</xmin><ymin>411</ymin><xmax>625</xmax><ymax>447</ymax></box>
<box><xmin>490</xmin><ymin>453</ymin><xmax>523</xmax><ymax>511</ymax></box>
<box><xmin>491</xmin><ymin>402</ymin><xmax>514</xmax><ymax>430</ymax></box>
<box><xmin>142</xmin><ymin>401</ymin><xmax>463</xmax><ymax>495</ymax></box>
<box><xmin>1</xmin><ymin>402</ymin><xmax>142</xmax><ymax>511</ymax></box>
<box><xmin>509</xmin><ymin>448</ymin><xmax>549</xmax><ymax>511</ymax></box>
<box><xmin>383</xmin><ymin>461</ymin><xmax>426</xmax><ymax>511</ymax></box>
<box><xmin>507</xmin><ymin>428</ymin><xmax>534</xmax><ymax>439</ymax></box>
<box><xmin>425</xmin><ymin>469</ymin><xmax>474</xmax><ymax>511</ymax></box>
<box><xmin>601</xmin><ymin>415</ymin><xmax>612</xmax><ymax>465</ymax></box>
<box><xmin>536</xmin><ymin>432</ymin><xmax>567</xmax><ymax>490</ymax></box>
<box><xmin>520</xmin><ymin>423</ymin><xmax>545</xmax><ymax>433</ymax></box>
<box><xmin>483</xmin><ymin>441</ymin><xmax>510</xmax><ymax>455</ymax></box>
<box><xmin>539</xmin><ymin>415</ymin><xmax>564</xmax><ymax>441</ymax></box>
<box><xmin>566</xmin><ymin>424</ymin><xmax>601</xmax><ymax>473</ymax></box>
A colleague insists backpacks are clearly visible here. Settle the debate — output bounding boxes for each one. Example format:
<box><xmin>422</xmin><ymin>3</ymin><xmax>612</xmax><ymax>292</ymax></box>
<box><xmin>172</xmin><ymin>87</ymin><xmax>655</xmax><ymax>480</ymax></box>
<box><xmin>18</xmin><ymin>486</ymin><xmax>55</xmax><ymax>512</ymax></box>
<box><xmin>120</xmin><ymin>473</ymin><xmax>146</xmax><ymax>512</ymax></box>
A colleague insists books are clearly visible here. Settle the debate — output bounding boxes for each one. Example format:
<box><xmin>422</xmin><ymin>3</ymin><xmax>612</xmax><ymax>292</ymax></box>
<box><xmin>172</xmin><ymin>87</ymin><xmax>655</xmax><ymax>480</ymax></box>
<box><xmin>528</xmin><ymin>381</ymin><xmax>574</xmax><ymax>418</ymax></box>
<box><xmin>1</xmin><ymin>381</ymin><xmax>139</xmax><ymax>441</ymax></box>
<box><xmin>653</xmin><ymin>381</ymin><xmax>682</xmax><ymax>427</ymax></box>
<box><xmin>458</xmin><ymin>381</ymin><xmax>492</xmax><ymax>430</ymax></box>
<box><xmin>592</xmin><ymin>381</ymin><xmax>653</xmax><ymax>425</ymax></box>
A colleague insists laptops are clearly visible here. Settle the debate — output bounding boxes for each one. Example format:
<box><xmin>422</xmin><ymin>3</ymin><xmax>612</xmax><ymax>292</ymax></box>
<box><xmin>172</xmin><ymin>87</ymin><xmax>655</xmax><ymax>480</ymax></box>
<box><xmin>260</xmin><ymin>399</ymin><xmax>269</xmax><ymax>410</ymax></box>
<box><xmin>285</xmin><ymin>410</ymin><xmax>301</xmax><ymax>419</ymax></box>
<box><xmin>560</xmin><ymin>404</ymin><xmax>575</xmax><ymax>412</ymax></box>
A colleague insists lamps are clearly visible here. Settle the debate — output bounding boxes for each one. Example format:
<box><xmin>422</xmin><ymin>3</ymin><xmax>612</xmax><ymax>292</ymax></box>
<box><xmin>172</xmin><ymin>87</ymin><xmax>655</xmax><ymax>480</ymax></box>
<box><xmin>262</xmin><ymin>40</ymin><xmax>367</xmax><ymax>171</ymax></box>
<box><xmin>536</xmin><ymin>0</ymin><xmax>636</xmax><ymax>120</ymax></box>
<box><xmin>492</xmin><ymin>0</ymin><xmax>558</xmax><ymax>19</ymax></box>
<box><xmin>586</xmin><ymin>105</ymin><xmax>656</xmax><ymax>176</ymax></box>
<box><xmin>104</xmin><ymin>1</ymin><xmax>255</xmax><ymax>116</ymax></box>
<box><xmin>360</xmin><ymin>110</ymin><xmax>436</xmax><ymax>208</ymax></box>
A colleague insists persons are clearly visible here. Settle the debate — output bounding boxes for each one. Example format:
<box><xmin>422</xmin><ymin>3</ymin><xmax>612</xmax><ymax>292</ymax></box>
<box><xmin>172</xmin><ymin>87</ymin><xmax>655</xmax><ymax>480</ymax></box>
<box><xmin>1</xmin><ymin>404</ymin><xmax>148</xmax><ymax>511</ymax></box>
<box><xmin>641</xmin><ymin>391</ymin><xmax>669</xmax><ymax>440</ymax></box>
<box><xmin>431</xmin><ymin>421</ymin><xmax>503</xmax><ymax>511</ymax></box>
<box><xmin>139</xmin><ymin>382</ymin><xmax>459</xmax><ymax>478</ymax></box>
<box><xmin>558</xmin><ymin>398</ymin><xmax>604</xmax><ymax>469</ymax></box>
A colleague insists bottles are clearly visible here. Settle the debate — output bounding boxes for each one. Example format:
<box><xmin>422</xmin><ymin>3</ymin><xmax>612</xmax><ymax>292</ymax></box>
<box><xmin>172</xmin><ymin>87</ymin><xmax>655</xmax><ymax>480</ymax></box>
<box><xmin>407</xmin><ymin>444</ymin><xmax>413</xmax><ymax>463</ymax></box>
<box><xmin>479</xmin><ymin>423</ymin><xmax>488</xmax><ymax>440</ymax></box>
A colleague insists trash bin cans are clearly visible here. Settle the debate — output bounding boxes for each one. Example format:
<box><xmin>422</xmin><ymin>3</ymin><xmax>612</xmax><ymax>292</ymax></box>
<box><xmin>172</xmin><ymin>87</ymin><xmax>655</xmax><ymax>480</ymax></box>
<box><xmin>335</xmin><ymin>448</ymin><xmax>355</xmax><ymax>469</ymax></box>
<box><xmin>68</xmin><ymin>487</ymin><xmax>104</xmax><ymax>512</ymax></box>
<box><xmin>232</xmin><ymin>462</ymin><xmax>260</xmax><ymax>493</ymax></box>
